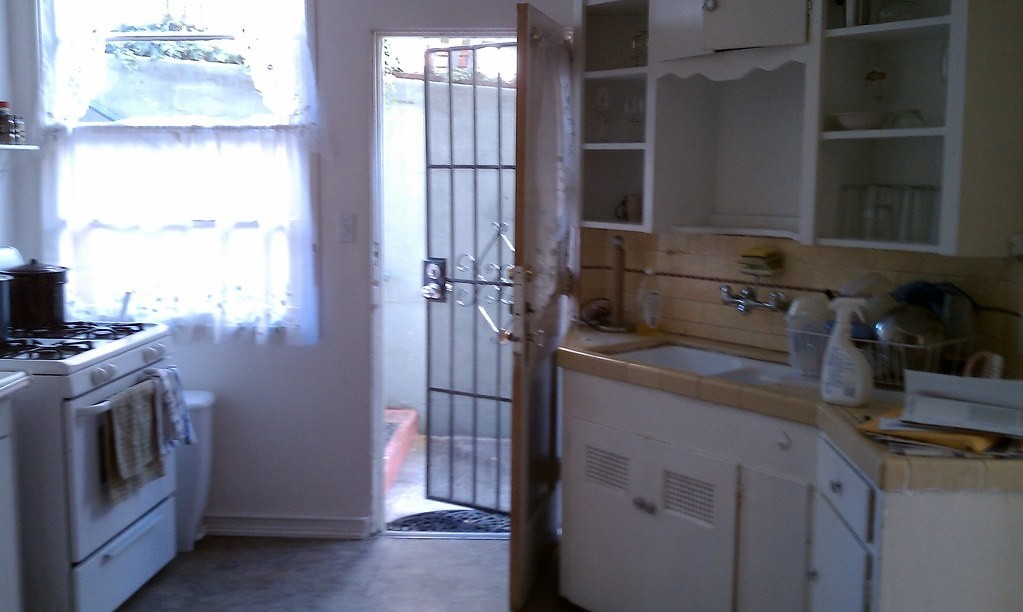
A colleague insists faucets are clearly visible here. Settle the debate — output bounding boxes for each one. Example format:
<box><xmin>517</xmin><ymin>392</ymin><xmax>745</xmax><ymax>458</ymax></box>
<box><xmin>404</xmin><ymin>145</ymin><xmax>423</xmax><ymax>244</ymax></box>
<box><xmin>734</xmin><ymin>287</ymin><xmax>762</xmax><ymax>319</ymax></box>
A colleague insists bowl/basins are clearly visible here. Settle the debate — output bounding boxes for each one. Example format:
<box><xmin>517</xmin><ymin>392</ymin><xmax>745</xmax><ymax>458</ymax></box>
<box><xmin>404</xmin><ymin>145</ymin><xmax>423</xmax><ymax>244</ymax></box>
<box><xmin>835</xmin><ymin>112</ymin><xmax>879</xmax><ymax>131</ymax></box>
<box><xmin>873</xmin><ymin>306</ymin><xmax>941</xmax><ymax>374</ymax></box>
<box><xmin>825</xmin><ymin>319</ymin><xmax>871</xmax><ymax>348</ymax></box>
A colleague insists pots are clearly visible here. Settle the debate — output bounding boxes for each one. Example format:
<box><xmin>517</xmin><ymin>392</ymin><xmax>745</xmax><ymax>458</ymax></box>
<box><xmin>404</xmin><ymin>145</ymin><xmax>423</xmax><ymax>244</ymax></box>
<box><xmin>2</xmin><ymin>258</ymin><xmax>68</xmax><ymax>331</ymax></box>
<box><xmin>0</xmin><ymin>275</ymin><xmax>14</xmax><ymax>327</ymax></box>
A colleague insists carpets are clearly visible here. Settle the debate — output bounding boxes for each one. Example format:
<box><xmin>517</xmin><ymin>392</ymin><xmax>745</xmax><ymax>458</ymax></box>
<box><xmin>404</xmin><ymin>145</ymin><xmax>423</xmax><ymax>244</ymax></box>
<box><xmin>387</xmin><ymin>508</ymin><xmax>514</xmax><ymax>534</ymax></box>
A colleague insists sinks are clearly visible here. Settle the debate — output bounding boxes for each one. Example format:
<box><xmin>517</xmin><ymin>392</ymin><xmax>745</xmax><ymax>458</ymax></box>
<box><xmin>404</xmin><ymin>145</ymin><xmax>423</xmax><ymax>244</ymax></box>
<box><xmin>592</xmin><ymin>336</ymin><xmax>792</xmax><ymax>393</ymax></box>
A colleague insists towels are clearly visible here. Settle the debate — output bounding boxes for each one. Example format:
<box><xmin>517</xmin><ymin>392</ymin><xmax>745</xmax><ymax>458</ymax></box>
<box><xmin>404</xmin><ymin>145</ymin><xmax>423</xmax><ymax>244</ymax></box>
<box><xmin>148</xmin><ymin>363</ymin><xmax>199</xmax><ymax>456</ymax></box>
<box><xmin>95</xmin><ymin>379</ymin><xmax>167</xmax><ymax>509</ymax></box>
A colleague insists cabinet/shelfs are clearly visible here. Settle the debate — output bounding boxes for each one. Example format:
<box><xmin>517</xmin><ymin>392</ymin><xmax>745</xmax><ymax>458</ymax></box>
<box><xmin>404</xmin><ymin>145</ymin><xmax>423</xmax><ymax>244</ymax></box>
<box><xmin>550</xmin><ymin>1</ymin><xmax>1023</xmax><ymax>611</ymax></box>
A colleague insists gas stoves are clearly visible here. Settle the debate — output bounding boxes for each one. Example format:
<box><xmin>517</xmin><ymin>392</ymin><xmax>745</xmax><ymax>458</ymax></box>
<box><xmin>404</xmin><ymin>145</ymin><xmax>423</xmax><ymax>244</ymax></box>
<box><xmin>0</xmin><ymin>320</ymin><xmax>158</xmax><ymax>363</ymax></box>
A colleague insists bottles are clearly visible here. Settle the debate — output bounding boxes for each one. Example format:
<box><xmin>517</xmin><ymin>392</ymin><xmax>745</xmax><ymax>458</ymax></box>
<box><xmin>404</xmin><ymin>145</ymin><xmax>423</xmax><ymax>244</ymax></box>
<box><xmin>638</xmin><ymin>268</ymin><xmax>662</xmax><ymax>337</ymax></box>
<box><xmin>14</xmin><ymin>114</ymin><xmax>25</xmax><ymax>145</ymax></box>
<box><xmin>0</xmin><ymin>100</ymin><xmax>16</xmax><ymax>145</ymax></box>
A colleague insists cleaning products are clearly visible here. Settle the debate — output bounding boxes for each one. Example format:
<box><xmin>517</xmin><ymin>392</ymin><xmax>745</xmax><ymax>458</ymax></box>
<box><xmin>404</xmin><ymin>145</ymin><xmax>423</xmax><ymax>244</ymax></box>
<box><xmin>818</xmin><ymin>295</ymin><xmax>875</xmax><ymax>410</ymax></box>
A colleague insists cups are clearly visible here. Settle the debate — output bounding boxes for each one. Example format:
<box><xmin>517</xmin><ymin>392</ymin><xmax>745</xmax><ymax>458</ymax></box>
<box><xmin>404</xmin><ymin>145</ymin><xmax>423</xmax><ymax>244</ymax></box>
<box><xmin>613</xmin><ymin>195</ymin><xmax>642</xmax><ymax>224</ymax></box>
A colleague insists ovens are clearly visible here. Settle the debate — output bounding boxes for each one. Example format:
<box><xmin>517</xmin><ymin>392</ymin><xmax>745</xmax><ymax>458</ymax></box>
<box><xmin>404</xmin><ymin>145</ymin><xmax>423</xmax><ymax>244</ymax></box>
<box><xmin>2</xmin><ymin>323</ymin><xmax>179</xmax><ymax>612</ymax></box>
